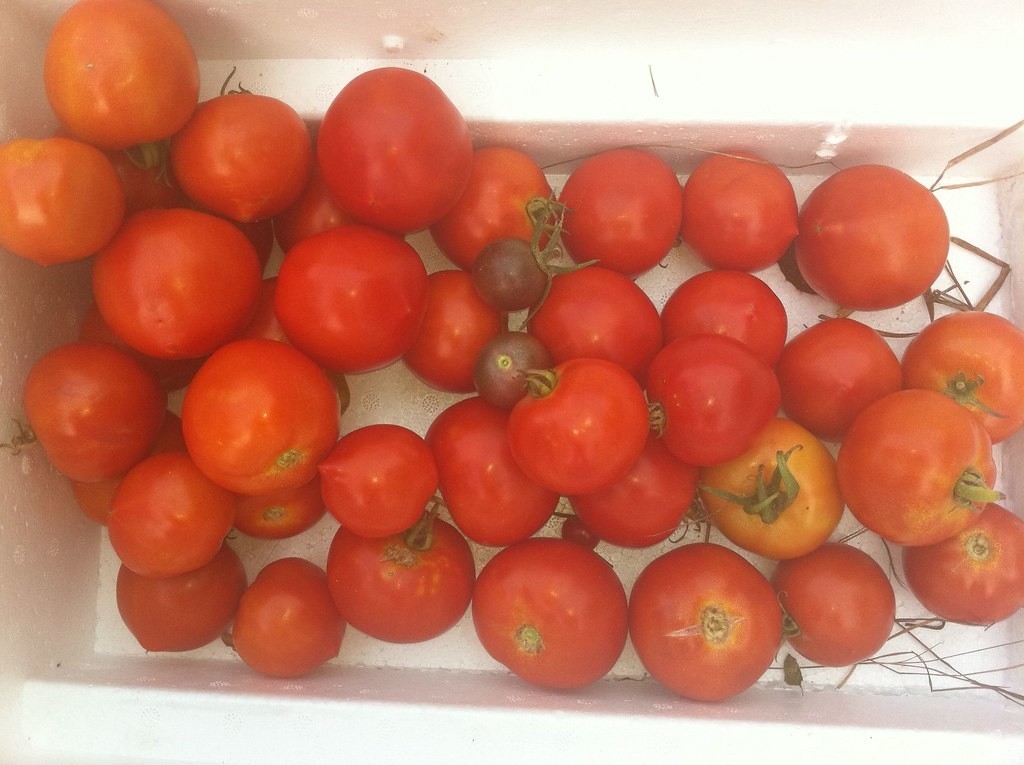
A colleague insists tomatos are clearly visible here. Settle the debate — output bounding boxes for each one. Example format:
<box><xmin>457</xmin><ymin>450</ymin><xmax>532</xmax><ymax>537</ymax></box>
<box><xmin>0</xmin><ymin>0</ymin><xmax>1024</xmax><ymax>699</ymax></box>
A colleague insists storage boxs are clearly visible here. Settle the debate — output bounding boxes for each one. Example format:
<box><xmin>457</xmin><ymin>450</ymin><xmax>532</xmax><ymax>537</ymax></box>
<box><xmin>0</xmin><ymin>0</ymin><xmax>1024</xmax><ymax>765</ymax></box>
